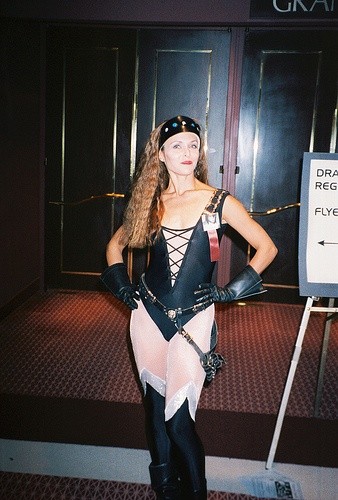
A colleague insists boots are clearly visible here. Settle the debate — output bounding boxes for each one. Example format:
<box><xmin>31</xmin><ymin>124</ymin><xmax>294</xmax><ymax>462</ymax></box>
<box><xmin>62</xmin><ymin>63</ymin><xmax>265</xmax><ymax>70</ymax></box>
<box><xmin>149</xmin><ymin>462</ymin><xmax>185</xmax><ymax>500</ymax></box>
<box><xmin>185</xmin><ymin>480</ymin><xmax>207</xmax><ymax>500</ymax></box>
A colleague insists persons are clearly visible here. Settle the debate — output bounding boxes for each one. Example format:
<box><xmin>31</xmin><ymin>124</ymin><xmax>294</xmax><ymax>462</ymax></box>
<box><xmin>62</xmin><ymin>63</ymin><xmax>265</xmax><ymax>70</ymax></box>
<box><xmin>104</xmin><ymin>114</ymin><xmax>279</xmax><ymax>500</ymax></box>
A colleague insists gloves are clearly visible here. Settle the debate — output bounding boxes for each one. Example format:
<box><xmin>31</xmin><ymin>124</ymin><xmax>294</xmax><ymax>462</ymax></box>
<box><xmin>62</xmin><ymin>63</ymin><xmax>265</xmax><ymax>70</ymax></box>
<box><xmin>194</xmin><ymin>265</ymin><xmax>268</xmax><ymax>310</ymax></box>
<box><xmin>99</xmin><ymin>263</ymin><xmax>139</xmax><ymax>310</ymax></box>
